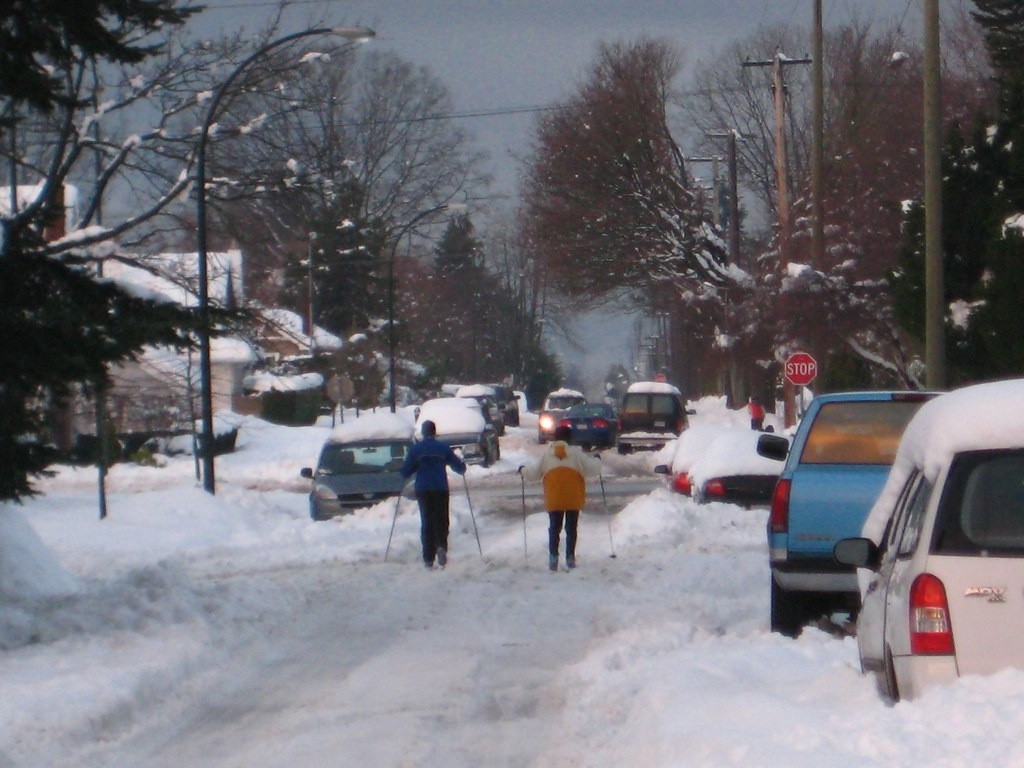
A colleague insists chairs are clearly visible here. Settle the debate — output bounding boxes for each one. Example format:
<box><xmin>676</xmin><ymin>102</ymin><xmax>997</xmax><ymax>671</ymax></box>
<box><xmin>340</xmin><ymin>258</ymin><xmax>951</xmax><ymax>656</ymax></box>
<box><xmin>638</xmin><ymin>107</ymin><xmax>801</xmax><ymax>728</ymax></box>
<box><xmin>385</xmin><ymin>445</ymin><xmax>408</xmax><ymax>473</ymax></box>
<box><xmin>959</xmin><ymin>456</ymin><xmax>1024</xmax><ymax>557</ymax></box>
<box><xmin>335</xmin><ymin>450</ymin><xmax>360</xmax><ymax>474</ymax></box>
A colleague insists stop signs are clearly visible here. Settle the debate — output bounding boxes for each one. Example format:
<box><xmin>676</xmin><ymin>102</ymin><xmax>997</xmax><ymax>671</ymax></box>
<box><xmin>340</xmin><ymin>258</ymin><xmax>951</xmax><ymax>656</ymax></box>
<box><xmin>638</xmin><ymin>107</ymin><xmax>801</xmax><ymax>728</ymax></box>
<box><xmin>784</xmin><ymin>352</ymin><xmax>817</xmax><ymax>386</ymax></box>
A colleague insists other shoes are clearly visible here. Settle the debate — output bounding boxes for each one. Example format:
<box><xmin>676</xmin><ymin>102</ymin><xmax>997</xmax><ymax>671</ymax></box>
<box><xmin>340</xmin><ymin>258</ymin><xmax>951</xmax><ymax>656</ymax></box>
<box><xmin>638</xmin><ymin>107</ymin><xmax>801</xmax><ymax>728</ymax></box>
<box><xmin>567</xmin><ymin>559</ymin><xmax>576</xmax><ymax>568</ymax></box>
<box><xmin>550</xmin><ymin>562</ymin><xmax>558</xmax><ymax>571</ymax></box>
<box><xmin>437</xmin><ymin>546</ymin><xmax>448</xmax><ymax>566</ymax></box>
<box><xmin>425</xmin><ymin>561</ymin><xmax>433</xmax><ymax>567</ymax></box>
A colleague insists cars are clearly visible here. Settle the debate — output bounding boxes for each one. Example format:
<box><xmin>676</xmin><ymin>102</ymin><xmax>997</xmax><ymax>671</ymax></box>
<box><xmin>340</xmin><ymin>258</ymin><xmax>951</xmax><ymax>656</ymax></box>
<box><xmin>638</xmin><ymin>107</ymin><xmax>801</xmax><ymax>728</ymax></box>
<box><xmin>831</xmin><ymin>446</ymin><xmax>1024</xmax><ymax>704</ymax></box>
<box><xmin>539</xmin><ymin>391</ymin><xmax>589</xmax><ymax>444</ymax></box>
<box><xmin>414</xmin><ymin>403</ymin><xmax>501</xmax><ymax>469</ymax></box>
<box><xmin>654</xmin><ymin>463</ymin><xmax>692</xmax><ymax>498</ymax></box>
<box><xmin>687</xmin><ymin>473</ymin><xmax>780</xmax><ymax>514</ymax></box>
<box><xmin>555</xmin><ymin>403</ymin><xmax>619</xmax><ymax>451</ymax></box>
<box><xmin>483</xmin><ymin>383</ymin><xmax>521</xmax><ymax>428</ymax></box>
<box><xmin>300</xmin><ymin>432</ymin><xmax>419</xmax><ymax>522</ymax></box>
<box><xmin>455</xmin><ymin>395</ymin><xmax>507</xmax><ymax>435</ymax></box>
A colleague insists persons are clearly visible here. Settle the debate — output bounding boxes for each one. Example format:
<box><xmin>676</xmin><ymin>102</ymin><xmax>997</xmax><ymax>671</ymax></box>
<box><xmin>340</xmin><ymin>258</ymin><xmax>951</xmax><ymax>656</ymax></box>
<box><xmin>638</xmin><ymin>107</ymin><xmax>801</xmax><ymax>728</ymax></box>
<box><xmin>400</xmin><ymin>420</ymin><xmax>467</xmax><ymax>570</ymax></box>
<box><xmin>748</xmin><ymin>398</ymin><xmax>766</xmax><ymax>431</ymax></box>
<box><xmin>518</xmin><ymin>425</ymin><xmax>602</xmax><ymax>571</ymax></box>
<box><xmin>384</xmin><ymin>444</ymin><xmax>405</xmax><ymax>472</ymax></box>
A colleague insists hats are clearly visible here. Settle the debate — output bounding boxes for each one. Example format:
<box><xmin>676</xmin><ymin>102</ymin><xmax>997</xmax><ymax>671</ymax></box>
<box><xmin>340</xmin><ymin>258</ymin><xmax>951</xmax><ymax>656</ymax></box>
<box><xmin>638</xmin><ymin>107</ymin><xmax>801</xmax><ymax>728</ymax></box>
<box><xmin>422</xmin><ymin>421</ymin><xmax>436</xmax><ymax>436</ymax></box>
<box><xmin>555</xmin><ymin>426</ymin><xmax>572</xmax><ymax>441</ymax></box>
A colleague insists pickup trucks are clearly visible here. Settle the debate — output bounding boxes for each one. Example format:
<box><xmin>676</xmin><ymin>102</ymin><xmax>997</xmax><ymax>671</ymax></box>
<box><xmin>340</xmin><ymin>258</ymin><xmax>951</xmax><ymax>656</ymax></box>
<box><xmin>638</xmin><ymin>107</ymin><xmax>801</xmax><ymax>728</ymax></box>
<box><xmin>755</xmin><ymin>390</ymin><xmax>952</xmax><ymax>640</ymax></box>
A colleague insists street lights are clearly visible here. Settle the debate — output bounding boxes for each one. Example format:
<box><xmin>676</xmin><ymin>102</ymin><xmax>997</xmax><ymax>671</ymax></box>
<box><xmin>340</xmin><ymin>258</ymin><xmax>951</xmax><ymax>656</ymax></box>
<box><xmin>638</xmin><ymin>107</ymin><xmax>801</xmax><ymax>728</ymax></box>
<box><xmin>387</xmin><ymin>202</ymin><xmax>470</xmax><ymax>415</ymax></box>
<box><xmin>196</xmin><ymin>25</ymin><xmax>377</xmax><ymax>495</ymax></box>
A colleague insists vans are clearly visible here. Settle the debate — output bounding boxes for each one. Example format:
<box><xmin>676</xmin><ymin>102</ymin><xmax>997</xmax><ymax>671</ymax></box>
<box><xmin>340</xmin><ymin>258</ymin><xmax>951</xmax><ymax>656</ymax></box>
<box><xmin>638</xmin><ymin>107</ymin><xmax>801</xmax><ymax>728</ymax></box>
<box><xmin>616</xmin><ymin>392</ymin><xmax>697</xmax><ymax>455</ymax></box>
